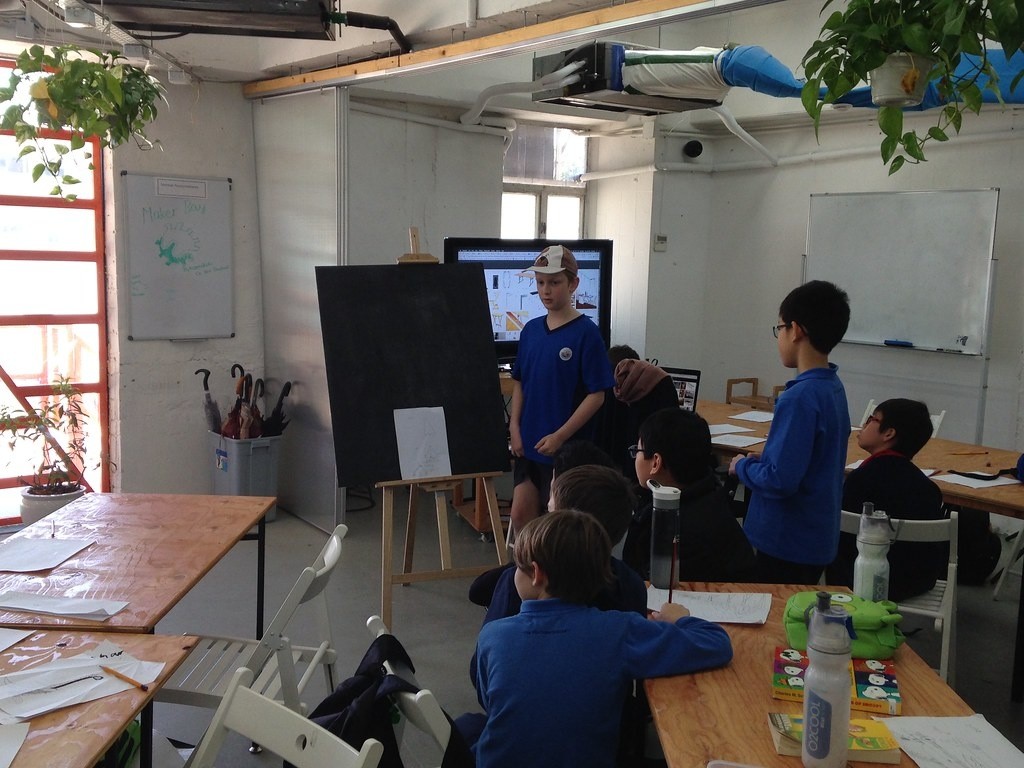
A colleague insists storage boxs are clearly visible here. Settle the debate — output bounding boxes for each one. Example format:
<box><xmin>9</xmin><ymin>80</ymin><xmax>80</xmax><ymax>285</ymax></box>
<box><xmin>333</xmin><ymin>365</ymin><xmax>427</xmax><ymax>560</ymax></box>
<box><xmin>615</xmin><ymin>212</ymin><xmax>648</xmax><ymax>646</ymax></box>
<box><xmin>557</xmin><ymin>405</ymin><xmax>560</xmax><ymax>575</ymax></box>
<box><xmin>208</xmin><ymin>429</ymin><xmax>284</xmax><ymax>524</ymax></box>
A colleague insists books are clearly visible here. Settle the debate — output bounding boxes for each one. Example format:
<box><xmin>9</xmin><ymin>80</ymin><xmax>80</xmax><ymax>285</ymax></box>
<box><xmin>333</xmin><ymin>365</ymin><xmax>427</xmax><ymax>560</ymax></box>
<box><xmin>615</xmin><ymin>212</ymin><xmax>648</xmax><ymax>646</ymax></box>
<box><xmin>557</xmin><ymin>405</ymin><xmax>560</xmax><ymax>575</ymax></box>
<box><xmin>773</xmin><ymin>644</ymin><xmax>902</xmax><ymax>716</ymax></box>
<box><xmin>767</xmin><ymin>712</ymin><xmax>901</xmax><ymax>764</ymax></box>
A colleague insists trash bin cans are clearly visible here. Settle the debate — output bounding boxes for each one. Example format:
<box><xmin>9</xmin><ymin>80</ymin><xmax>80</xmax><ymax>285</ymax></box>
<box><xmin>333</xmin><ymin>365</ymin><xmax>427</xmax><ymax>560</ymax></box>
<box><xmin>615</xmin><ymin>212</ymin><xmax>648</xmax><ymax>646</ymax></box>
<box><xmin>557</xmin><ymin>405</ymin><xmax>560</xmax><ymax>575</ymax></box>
<box><xmin>206</xmin><ymin>429</ymin><xmax>285</xmax><ymax>523</ymax></box>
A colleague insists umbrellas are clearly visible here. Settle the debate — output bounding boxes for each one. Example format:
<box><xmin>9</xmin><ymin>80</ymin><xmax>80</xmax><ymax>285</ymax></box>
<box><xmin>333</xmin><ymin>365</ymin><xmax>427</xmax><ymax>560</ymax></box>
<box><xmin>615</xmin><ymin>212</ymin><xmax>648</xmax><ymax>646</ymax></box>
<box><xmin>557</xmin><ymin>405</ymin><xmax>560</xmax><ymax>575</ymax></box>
<box><xmin>195</xmin><ymin>369</ymin><xmax>222</xmax><ymax>434</ymax></box>
<box><xmin>260</xmin><ymin>381</ymin><xmax>292</xmax><ymax>437</ymax></box>
<box><xmin>222</xmin><ymin>363</ymin><xmax>265</xmax><ymax>440</ymax></box>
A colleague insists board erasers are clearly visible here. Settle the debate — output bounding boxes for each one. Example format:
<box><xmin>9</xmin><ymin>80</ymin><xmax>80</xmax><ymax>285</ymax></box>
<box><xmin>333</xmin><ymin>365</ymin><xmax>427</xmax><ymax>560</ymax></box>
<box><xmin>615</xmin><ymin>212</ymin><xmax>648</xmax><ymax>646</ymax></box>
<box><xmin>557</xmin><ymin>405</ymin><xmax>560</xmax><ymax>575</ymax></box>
<box><xmin>884</xmin><ymin>339</ymin><xmax>914</xmax><ymax>349</ymax></box>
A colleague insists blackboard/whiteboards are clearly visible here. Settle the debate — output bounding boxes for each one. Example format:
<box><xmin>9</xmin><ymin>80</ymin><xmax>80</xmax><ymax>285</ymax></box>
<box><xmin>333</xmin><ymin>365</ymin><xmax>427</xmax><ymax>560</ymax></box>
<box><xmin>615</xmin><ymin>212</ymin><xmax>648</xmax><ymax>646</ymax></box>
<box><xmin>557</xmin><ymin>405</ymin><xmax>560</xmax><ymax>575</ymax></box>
<box><xmin>314</xmin><ymin>260</ymin><xmax>512</xmax><ymax>488</ymax></box>
<box><xmin>121</xmin><ymin>170</ymin><xmax>236</xmax><ymax>340</ymax></box>
<box><xmin>803</xmin><ymin>186</ymin><xmax>1002</xmax><ymax>357</ymax></box>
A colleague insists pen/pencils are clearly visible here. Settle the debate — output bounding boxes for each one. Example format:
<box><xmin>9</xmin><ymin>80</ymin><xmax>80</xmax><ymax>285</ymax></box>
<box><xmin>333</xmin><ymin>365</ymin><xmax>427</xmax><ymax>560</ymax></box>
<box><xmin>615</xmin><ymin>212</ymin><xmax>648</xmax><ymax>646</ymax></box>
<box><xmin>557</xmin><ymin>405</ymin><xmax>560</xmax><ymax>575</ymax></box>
<box><xmin>51</xmin><ymin>520</ymin><xmax>55</xmax><ymax>539</ymax></box>
<box><xmin>929</xmin><ymin>470</ymin><xmax>942</xmax><ymax>476</ymax></box>
<box><xmin>99</xmin><ymin>665</ymin><xmax>148</xmax><ymax>693</ymax></box>
<box><xmin>952</xmin><ymin>452</ymin><xmax>988</xmax><ymax>456</ymax></box>
<box><xmin>667</xmin><ymin>534</ymin><xmax>678</xmax><ymax>610</ymax></box>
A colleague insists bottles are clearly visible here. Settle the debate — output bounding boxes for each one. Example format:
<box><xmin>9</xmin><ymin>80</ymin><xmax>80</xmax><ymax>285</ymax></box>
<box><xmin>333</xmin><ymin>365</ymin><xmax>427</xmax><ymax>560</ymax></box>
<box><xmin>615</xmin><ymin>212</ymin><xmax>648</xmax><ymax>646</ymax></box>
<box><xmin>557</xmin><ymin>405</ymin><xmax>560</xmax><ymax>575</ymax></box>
<box><xmin>853</xmin><ymin>503</ymin><xmax>893</xmax><ymax>602</ymax></box>
<box><xmin>647</xmin><ymin>479</ymin><xmax>681</xmax><ymax>589</ymax></box>
<box><xmin>801</xmin><ymin>592</ymin><xmax>854</xmax><ymax>768</ymax></box>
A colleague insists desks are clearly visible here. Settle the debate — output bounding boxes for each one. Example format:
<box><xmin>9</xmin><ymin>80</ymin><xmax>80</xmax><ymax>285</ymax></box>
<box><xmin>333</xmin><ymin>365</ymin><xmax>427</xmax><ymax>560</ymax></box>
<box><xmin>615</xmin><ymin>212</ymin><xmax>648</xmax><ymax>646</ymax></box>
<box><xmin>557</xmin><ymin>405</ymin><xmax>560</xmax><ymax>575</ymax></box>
<box><xmin>0</xmin><ymin>492</ymin><xmax>277</xmax><ymax>766</ymax></box>
<box><xmin>643</xmin><ymin>581</ymin><xmax>1024</xmax><ymax>768</ymax></box>
<box><xmin>694</xmin><ymin>402</ymin><xmax>1024</xmax><ymax>703</ymax></box>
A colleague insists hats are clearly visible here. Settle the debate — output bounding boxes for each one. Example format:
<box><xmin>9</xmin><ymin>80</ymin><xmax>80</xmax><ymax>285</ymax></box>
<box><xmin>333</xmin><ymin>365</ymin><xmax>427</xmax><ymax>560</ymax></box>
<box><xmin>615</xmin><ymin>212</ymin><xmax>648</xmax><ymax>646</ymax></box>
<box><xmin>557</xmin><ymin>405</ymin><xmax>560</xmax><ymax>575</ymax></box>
<box><xmin>522</xmin><ymin>245</ymin><xmax>577</xmax><ymax>273</ymax></box>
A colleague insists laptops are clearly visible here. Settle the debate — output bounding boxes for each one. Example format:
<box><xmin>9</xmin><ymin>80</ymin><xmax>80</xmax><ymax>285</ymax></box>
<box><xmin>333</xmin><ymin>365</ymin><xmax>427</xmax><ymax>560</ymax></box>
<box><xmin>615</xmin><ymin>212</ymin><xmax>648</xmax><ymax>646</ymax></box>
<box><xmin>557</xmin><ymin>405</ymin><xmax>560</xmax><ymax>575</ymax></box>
<box><xmin>659</xmin><ymin>366</ymin><xmax>701</xmax><ymax>413</ymax></box>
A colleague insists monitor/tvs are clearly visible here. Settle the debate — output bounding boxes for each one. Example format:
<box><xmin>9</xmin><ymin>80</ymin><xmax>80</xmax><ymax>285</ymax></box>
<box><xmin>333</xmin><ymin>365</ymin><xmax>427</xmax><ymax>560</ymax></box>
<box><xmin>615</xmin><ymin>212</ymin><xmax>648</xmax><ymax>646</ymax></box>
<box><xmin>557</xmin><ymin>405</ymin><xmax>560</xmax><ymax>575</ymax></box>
<box><xmin>444</xmin><ymin>236</ymin><xmax>613</xmax><ymax>365</ymax></box>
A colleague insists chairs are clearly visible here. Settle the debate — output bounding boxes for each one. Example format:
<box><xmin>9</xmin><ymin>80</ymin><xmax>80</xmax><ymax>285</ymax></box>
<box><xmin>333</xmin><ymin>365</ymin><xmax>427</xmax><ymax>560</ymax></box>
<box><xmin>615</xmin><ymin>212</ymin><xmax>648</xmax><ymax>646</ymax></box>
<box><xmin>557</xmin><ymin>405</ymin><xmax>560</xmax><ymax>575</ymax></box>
<box><xmin>819</xmin><ymin>511</ymin><xmax>1024</xmax><ymax>691</ymax></box>
<box><xmin>128</xmin><ymin>524</ymin><xmax>449</xmax><ymax>768</ymax></box>
<box><xmin>860</xmin><ymin>399</ymin><xmax>945</xmax><ymax>438</ymax></box>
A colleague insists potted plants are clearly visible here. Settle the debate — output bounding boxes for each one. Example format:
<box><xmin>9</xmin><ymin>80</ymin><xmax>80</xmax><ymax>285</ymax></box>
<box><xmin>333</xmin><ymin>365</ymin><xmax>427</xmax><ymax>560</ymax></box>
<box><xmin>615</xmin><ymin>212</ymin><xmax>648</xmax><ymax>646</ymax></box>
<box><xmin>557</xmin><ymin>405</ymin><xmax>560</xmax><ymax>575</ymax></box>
<box><xmin>0</xmin><ymin>375</ymin><xmax>117</xmax><ymax>525</ymax></box>
<box><xmin>0</xmin><ymin>45</ymin><xmax>170</xmax><ymax>202</ymax></box>
<box><xmin>798</xmin><ymin>0</ymin><xmax>1024</xmax><ymax>176</ymax></box>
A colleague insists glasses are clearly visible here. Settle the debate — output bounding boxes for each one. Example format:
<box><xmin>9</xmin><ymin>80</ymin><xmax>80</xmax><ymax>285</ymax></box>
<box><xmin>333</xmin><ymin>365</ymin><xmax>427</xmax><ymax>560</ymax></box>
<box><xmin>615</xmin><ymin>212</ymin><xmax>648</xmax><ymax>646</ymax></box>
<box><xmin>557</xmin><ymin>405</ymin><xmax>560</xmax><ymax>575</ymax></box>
<box><xmin>865</xmin><ymin>414</ymin><xmax>892</xmax><ymax>428</ymax></box>
<box><xmin>628</xmin><ymin>445</ymin><xmax>655</xmax><ymax>458</ymax></box>
<box><xmin>773</xmin><ymin>324</ymin><xmax>807</xmax><ymax>339</ymax></box>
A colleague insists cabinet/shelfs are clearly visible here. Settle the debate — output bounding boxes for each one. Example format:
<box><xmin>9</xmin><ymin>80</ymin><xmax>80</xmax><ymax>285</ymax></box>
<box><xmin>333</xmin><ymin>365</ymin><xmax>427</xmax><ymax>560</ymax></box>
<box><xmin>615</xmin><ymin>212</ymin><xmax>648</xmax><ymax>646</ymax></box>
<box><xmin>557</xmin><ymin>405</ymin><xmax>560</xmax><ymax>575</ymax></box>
<box><xmin>452</xmin><ymin>372</ymin><xmax>515</xmax><ymax>543</ymax></box>
<box><xmin>726</xmin><ymin>378</ymin><xmax>785</xmax><ymax>413</ymax></box>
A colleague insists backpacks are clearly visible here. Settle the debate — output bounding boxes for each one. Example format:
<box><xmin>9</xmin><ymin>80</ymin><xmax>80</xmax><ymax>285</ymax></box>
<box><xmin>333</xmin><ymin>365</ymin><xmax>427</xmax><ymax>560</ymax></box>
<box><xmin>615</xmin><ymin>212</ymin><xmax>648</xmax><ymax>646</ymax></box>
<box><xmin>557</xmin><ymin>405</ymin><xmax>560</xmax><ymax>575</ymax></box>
<box><xmin>939</xmin><ymin>502</ymin><xmax>1002</xmax><ymax>587</ymax></box>
<box><xmin>283</xmin><ymin>634</ymin><xmax>460</xmax><ymax>768</ymax></box>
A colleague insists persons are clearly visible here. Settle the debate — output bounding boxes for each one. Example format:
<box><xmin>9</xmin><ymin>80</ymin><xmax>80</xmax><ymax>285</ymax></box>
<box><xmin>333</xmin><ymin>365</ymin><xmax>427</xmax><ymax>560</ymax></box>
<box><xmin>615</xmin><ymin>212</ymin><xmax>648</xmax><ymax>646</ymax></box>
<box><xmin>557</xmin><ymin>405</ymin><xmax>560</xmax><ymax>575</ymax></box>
<box><xmin>608</xmin><ymin>346</ymin><xmax>640</xmax><ymax>375</ymax></box>
<box><xmin>470</xmin><ymin>509</ymin><xmax>734</xmax><ymax>768</ymax></box>
<box><xmin>819</xmin><ymin>397</ymin><xmax>951</xmax><ymax>602</ymax></box>
<box><xmin>471</xmin><ymin>464</ymin><xmax>647</xmax><ymax>618</ymax></box>
<box><xmin>510</xmin><ymin>244</ymin><xmax>618</xmax><ymax>539</ymax></box>
<box><xmin>729</xmin><ymin>280</ymin><xmax>852</xmax><ymax>584</ymax></box>
<box><xmin>622</xmin><ymin>406</ymin><xmax>757</xmax><ymax>584</ymax></box>
